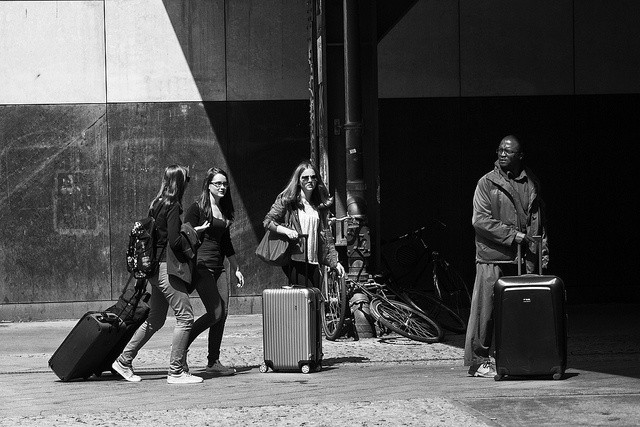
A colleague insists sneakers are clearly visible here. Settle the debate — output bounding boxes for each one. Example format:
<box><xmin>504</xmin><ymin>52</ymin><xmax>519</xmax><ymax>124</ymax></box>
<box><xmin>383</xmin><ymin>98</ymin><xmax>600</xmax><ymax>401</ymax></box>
<box><xmin>206</xmin><ymin>359</ymin><xmax>236</xmax><ymax>375</ymax></box>
<box><xmin>112</xmin><ymin>359</ymin><xmax>142</xmax><ymax>382</ymax></box>
<box><xmin>167</xmin><ymin>370</ymin><xmax>204</xmax><ymax>383</ymax></box>
<box><xmin>468</xmin><ymin>363</ymin><xmax>495</xmax><ymax>377</ymax></box>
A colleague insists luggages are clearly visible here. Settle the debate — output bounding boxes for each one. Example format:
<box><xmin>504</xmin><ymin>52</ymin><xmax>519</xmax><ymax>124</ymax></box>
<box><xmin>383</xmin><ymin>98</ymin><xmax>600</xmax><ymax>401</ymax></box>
<box><xmin>105</xmin><ymin>280</ymin><xmax>149</xmax><ymax>368</ymax></box>
<box><xmin>48</xmin><ymin>288</ymin><xmax>144</xmax><ymax>381</ymax></box>
<box><xmin>494</xmin><ymin>235</ymin><xmax>568</xmax><ymax>380</ymax></box>
<box><xmin>259</xmin><ymin>234</ymin><xmax>323</xmax><ymax>374</ymax></box>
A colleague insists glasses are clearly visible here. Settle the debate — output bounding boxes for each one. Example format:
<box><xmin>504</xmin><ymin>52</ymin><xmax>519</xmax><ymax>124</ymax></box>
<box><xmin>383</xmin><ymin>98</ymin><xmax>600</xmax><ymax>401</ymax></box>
<box><xmin>298</xmin><ymin>178</ymin><xmax>318</xmax><ymax>185</ymax></box>
<box><xmin>210</xmin><ymin>181</ymin><xmax>229</xmax><ymax>186</ymax></box>
<box><xmin>496</xmin><ymin>147</ymin><xmax>519</xmax><ymax>154</ymax></box>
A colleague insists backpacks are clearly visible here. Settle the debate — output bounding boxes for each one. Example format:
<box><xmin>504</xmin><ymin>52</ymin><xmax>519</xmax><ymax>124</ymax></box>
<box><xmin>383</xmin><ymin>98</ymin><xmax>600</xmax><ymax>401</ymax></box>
<box><xmin>126</xmin><ymin>195</ymin><xmax>169</xmax><ymax>278</ymax></box>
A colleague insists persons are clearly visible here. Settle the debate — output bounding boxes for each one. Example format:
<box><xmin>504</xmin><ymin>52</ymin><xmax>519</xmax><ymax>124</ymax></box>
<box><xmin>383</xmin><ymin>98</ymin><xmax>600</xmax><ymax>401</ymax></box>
<box><xmin>184</xmin><ymin>166</ymin><xmax>245</xmax><ymax>375</ymax></box>
<box><xmin>463</xmin><ymin>135</ymin><xmax>551</xmax><ymax>379</ymax></box>
<box><xmin>262</xmin><ymin>162</ymin><xmax>344</xmax><ymax>290</ymax></box>
<box><xmin>111</xmin><ymin>163</ymin><xmax>211</xmax><ymax>384</ymax></box>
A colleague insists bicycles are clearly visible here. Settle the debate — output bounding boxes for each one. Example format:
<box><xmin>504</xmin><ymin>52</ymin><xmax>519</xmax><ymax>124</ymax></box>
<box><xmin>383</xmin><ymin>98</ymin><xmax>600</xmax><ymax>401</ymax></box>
<box><xmin>319</xmin><ymin>211</ymin><xmax>355</xmax><ymax>341</ymax></box>
<box><xmin>345</xmin><ymin>271</ymin><xmax>466</xmax><ymax>343</ymax></box>
<box><xmin>382</xmin><ymin>220</ymin><xmax>471</xmax><ymax>324</ymax></box>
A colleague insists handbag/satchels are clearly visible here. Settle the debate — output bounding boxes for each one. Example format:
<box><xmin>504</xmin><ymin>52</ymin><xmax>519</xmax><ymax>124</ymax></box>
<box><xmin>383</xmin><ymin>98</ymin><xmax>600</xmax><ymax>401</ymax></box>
<box><xmin>255</xmin><ymin>195</ymin><xmax>292</xmax><ymax>266</ymax></box>
